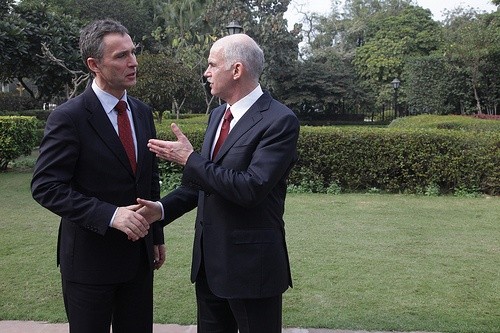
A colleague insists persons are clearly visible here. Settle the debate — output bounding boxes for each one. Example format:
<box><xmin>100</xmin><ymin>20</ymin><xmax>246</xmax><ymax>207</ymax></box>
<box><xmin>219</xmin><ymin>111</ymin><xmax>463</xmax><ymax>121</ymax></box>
<box><xmin>128</xmin><ymin>34</ymin><xmax>300</xmax><ymax>333</ymax></box>
<box><xmin>30</xmin><ymin>20</ymin><xmax>165</xmax><ymax>333</ymax></box>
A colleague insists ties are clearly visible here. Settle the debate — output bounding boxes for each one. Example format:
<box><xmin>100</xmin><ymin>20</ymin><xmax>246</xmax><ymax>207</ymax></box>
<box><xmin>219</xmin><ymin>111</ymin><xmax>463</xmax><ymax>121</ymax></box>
<box><xmin>211</xmin><ymin>107</ymin><xmax>233</xmax><ymax>162</ymax></box>
<box><xmin>114</xmin><ymin>101</ymin><xmax>136</xmax><ymax>174</ymax></box>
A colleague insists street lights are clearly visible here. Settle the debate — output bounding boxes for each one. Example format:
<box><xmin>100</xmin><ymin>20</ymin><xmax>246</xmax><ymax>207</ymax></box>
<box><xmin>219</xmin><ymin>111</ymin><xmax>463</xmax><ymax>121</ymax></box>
<box><xmin>225</xmin><ymin>21</ymin><xmax>244</xmax><ymax>34</ymax></box>
<box><xmin>391</xmin><ymin>78</ymin><xmax>401</xmax><ymax>120</ymax></box>
